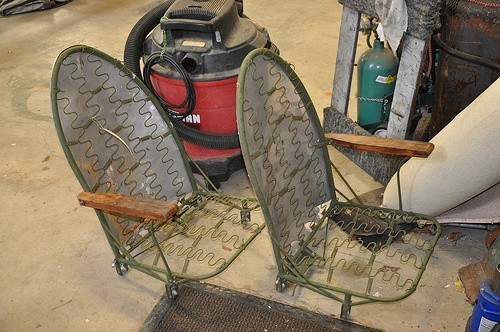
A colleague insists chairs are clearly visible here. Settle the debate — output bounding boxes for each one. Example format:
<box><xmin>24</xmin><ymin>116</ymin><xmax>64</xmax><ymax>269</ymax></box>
<box><xmin>49</xmin><ymin>45</ymin><xmax>273</xmax><ymax>302</ymax></box>
<box><xmin>233</xmin><ymin>48</ymin><xmax>440</xmax><ymax>318</ymax></box>
